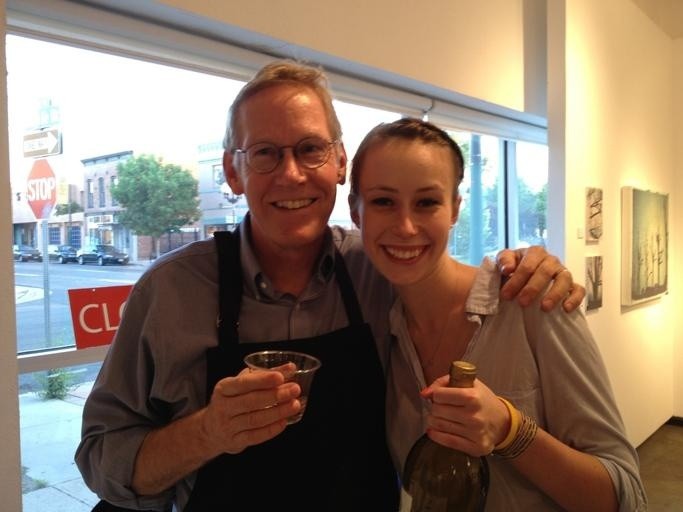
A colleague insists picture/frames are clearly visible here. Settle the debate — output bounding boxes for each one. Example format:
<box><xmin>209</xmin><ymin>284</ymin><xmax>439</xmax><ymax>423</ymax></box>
<box><xmin>622</xmin><ymin>185</ymin><xmax>670</xmax><ymax>307</ymax></box>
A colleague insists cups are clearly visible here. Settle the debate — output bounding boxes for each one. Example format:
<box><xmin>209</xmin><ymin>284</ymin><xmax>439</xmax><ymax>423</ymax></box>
<box><xmin>243</xmin><ymin>350</ymin><xmax>322</xmax><ymax>425</ymax></box>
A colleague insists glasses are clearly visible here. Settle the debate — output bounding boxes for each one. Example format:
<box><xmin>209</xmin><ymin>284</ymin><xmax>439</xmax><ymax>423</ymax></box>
<box><xmin>232</xmin><ymin>136</ymin><xmax>339</xmax><ymax>173</ymax></box>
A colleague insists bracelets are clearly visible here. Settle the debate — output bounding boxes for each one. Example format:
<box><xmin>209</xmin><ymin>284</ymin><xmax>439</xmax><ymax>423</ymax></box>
<box><xmin>493</xmin><ymin>412</ymin><xmax>537</xmax><ymax>463</ymax></box>
<box><xmin>491</xmin><ymin>395</ymin><xmax>518</xmax><ymax>452</ymax></box>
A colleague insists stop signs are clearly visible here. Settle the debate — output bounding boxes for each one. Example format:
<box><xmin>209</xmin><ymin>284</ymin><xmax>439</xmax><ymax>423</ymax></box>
<box><xmin>26</xmin><ymin>158</ymin><xmax>58</xmax><ymax>220</ymax></box>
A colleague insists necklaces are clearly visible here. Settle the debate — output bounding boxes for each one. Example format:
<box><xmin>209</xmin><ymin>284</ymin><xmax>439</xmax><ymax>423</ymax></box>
<box><xmin>416</xmin><ymin>260</ymin><xmax>458</xmax><ymax>368</ymax></box>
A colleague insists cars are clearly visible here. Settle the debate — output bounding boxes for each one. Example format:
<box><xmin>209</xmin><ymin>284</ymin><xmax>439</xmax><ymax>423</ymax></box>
<box><xmin>39</xmin><ymin>244</ymin><xmax>77</xmax><ymax>264</ymax></box>
<box><xmin>13</xmin><ymin>244</ymin><xmax>39</xmax><ymax>262</ymax></box>
<box><xmin>75</xmin><ymin>243</ymin><xmax>129</xmax><ymax>266</ymax></box>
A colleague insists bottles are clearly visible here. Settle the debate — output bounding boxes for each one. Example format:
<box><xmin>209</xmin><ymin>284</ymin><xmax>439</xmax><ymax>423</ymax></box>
<box><xmin>397</xmin><ymin>361</ymin><xmax>489</xmax><ymax>511</ymax></box>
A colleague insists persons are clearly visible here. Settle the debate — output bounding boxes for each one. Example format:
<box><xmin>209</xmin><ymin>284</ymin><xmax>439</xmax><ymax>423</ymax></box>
<box><xmin>345</xmin><ymin>116</ymin><xmax>650</xmax><ymax>512</ymax></box>
<box><xmin>72</xmin><ymin>55</ymin><xmax>589</xmax><ymax>512</ymax></box>
